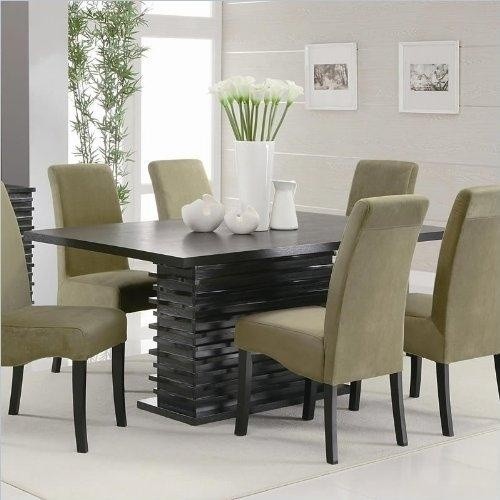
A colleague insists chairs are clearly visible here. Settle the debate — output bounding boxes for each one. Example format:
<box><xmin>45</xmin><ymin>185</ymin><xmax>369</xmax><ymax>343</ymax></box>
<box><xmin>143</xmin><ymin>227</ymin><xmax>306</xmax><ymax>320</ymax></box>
<box><xmin>47</xmin><ymin>164</ymin><xmax>158</xmax><ymax>373</ymax></box>
<box><xmin>233</xmin><ymin>193</ymin><xmax>430</xmax><ymax>465</ymax></box>
<box><xmin>348</xmin><ymin>182</ymin><xmax>500</xmax><ymax>438</ymax></box>
<box><xmin>148</xmin><ymin>158</ymin><xmax>214</xmax><ymax>220</ymax></box>
<box><xmin>344</xmin><ymin>158</ymin><xmax>418</xmax><ymax>217</ymax></box>
<box><xmin>1</xmin><ymin>178</ymin><xmax>127</xmax><ymax>453</ymax></box>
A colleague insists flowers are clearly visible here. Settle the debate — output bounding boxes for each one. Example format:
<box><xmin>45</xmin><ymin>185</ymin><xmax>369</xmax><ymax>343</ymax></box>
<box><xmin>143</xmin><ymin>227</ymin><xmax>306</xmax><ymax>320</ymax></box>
<box><xmin>209</xmin><ymin>74</ymin><xmax>303</xmax><ymax>145</ymax></box>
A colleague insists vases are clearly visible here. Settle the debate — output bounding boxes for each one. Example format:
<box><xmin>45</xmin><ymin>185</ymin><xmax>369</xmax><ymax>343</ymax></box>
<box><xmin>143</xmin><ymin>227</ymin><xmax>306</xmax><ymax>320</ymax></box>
<box><xmin>232</xmin><ymin>140</ymin><xmax>275</xmax><ymax>232</ymax></box>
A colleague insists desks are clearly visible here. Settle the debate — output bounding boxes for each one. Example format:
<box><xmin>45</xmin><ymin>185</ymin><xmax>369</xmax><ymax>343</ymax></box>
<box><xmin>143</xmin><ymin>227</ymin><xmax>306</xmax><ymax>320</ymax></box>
<box><xmin>23</xmin><ymin>212</ymin><xmax>443</xmax><ymax>426</ymax></box>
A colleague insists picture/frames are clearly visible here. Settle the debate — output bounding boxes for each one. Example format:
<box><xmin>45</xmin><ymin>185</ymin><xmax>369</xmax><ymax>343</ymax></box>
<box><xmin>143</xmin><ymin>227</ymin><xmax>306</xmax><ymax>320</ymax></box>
<box><xmin>304</xmin><ymin>42</ymin><xmax>358</xmax><ymax>112</ymax></box>
<box><xmin>397</xmin><ymin>39</ymin><xmax>460</xmax><ymax>114</ymax></box>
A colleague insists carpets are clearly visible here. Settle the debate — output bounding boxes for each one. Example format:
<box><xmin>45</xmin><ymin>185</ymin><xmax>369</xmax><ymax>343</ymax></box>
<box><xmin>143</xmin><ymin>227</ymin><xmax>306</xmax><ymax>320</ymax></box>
<box><xmin>0</xmin><ymin>354</ymin><xmax>499</xmax><ymax>500</ymax></box>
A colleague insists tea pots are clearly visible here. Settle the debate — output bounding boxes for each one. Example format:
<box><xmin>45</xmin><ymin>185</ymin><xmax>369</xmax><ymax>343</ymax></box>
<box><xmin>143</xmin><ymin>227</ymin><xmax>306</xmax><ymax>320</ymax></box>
<box><xmin>269</xmin><ymin>180</ymin><xmax>298</xmax><ymax>231</ymax></box>
